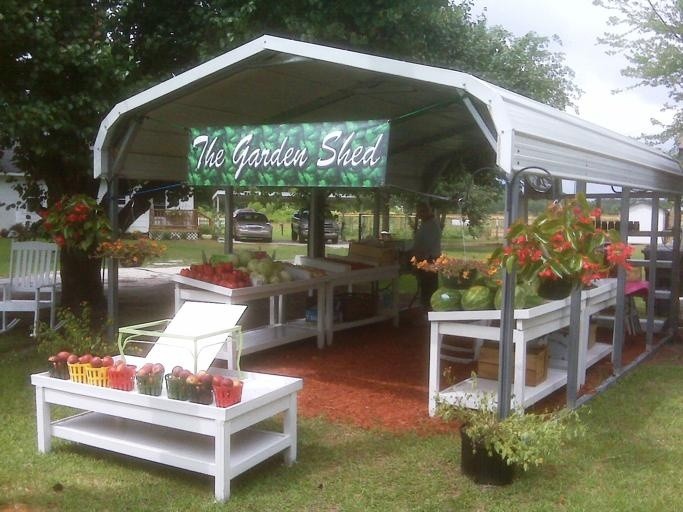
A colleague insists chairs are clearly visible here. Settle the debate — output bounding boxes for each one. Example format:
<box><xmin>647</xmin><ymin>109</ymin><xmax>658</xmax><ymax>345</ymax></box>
<box><xmin>0</xmin><ymin>238</ymin><xmax>59</xmax><ymax>337</ymax></box>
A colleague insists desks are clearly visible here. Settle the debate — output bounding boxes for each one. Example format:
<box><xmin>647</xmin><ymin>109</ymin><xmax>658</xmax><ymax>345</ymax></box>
<box><xmin>31</xmin><ymin>353</ymin><xmax>304</xmax><ymax>502</ymax></box>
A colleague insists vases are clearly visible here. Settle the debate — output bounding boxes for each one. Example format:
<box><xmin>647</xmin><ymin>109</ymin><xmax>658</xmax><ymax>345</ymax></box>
<box><xmin>538</xmin><ymin>280</ymin><xmax>572</xmax><ymax>300</ymax></box>
<box><xmin>441</xmin><ymin>268</ymin><xmax>477</xmax><ymax>290</ymax></box>
<box><xmin>119</xmin><ymin>255</ymin><xmax>145</xmax><ymax>268</ymax></box>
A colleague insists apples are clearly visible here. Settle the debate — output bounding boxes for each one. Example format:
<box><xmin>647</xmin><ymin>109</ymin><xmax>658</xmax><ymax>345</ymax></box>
<box><xmin>168</xmin><ymin>367</ymin><xmax>190</xmax><ymax>400</ymax></box>
<box><xmin>138</xmin><ymin>363</ymin><xmax>164</xmax><ymax>396</ymax></box>
<box><xmin>212</xmin><ymin>376</ymin><xmax>241</xmax><ymax>406</ymax></box>
<box><xmin>186</xmin><ymin>371</ymin><xmax>212</xmax><ymax>404</ymax></box>
<box><xmin>48</xmin><ymin>352</ymin><xmax>70</xmax><ymax>379</ymax></box>
<box><xmin>67</xmin><ymin>354</ymin><xmax>91</xmax><ymax>384</ymax></box>
<box><xmin>90</xmin><ymin>356</ymin><xmax>113</xmax><ymax>387</ymax></box>
<box><xmin>110</xmin><ymin>360</ymin><xmax>135</xmax><ymax>391</ymax></box>
<box><xmin>180</xmin><ymin>261</ymin><xmax>251</xmax><ymax>288</ymax></box>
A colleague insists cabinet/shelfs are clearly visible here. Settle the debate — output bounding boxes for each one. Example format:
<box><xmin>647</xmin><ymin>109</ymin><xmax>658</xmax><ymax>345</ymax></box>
<box><xmin>277</xmin><ymin>253</ymin><xmax>401</xmax><ymax>346</ymax></box>
<box><xmin>427</xmin><ymin>291</ymin><xmax>586</xmax><ymax>416</ymax></box>
<box><xmin>548</xmin><ymin>278</ymin><xmax>617</xmax><ymax>388</ymax></box>
<box><xmin>172</xmin><ymin>273</ymin><xmax>326</xmax><ymax>370</ymax></box>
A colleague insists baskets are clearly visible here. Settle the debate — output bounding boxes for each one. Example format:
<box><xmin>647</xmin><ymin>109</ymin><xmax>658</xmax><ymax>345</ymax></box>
<box><xmin>135</xmin><ymin>374</ymin><xmax>164</xmax><ymax>396</ymax></box>
<box><xmin>185</xmin><ymin>381</ymin><xmax>213</xmax><ymax>405</ymax></box>
<box><xmin>86</xmin><ymin>363</ymin><xmax>109</xmax><ymax>387</ymax></box>
<box><xmin>47</xmin><ymin>360</ymin><xmax>69</xmax><ymax>380</ymax></box>
<box><xmin>164</xmin><ymin>373</ymin><xmax>186</xmax><ymax>400</ymax></box>
<box><xmin>109</xmin><ymin>364</ymin><xmax>136</xmax><ymax>391</ymax></box>
<box><xmin>211</xmin><ymin>381</ymin><xmax>243</xmax><ymax>407</ymax></box>
<box><xmin>66</xmin><ymin>361</ymin><xmax>86</xmax><ymax>383</ymax></box>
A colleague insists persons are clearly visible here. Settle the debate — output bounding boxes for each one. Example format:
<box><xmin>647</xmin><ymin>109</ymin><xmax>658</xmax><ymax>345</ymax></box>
<box><xmin>412</xmin><ymin>203</ymin><xmax>440</xmax><ymax>310</ymax></box>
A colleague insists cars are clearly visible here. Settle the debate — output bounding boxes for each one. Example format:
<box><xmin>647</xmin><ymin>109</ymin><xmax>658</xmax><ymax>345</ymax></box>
<box><xmin>232</xmin><ymin>211</ymin><xmax>273</xmax><ymax>242</ymax></box>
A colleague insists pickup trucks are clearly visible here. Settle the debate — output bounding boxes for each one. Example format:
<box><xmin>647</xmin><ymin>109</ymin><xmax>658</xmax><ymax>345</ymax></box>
<box><xmin>291</xmin><ymin>208</ymin><xmax>339</xmax><ymax>244</ymax></box>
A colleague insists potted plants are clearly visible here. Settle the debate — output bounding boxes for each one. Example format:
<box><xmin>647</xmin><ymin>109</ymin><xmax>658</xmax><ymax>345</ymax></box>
<box><xmin>431</xmin><ymin>365</ymin><xmax>594</xmax><ymax>487</ymax></box>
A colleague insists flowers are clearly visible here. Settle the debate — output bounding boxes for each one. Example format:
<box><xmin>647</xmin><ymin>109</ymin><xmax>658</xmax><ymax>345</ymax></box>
<box><xmin>491</xmin><ymin>191</ymin><xmax>637</xmax><ymax>285</ymax></box>
<box><xmin>88</xmin><ymin>231</ymin><xmax>168</xmax><ymax>261</ymax></box>
<box><xmin>410</xmin><ymin>254</ymin><xmax>502</xmax><ymax>285</ymax></box>
<box><xmin>36</xmin><ymin>193</ymin><xmax>114</xmax><ymax>253</ymax></box>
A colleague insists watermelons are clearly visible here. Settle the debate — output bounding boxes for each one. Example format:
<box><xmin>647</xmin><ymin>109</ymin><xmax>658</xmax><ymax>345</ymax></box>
<box><xmin>517</xmin><ymin>283</ymin><xmax>538</xmax><ymax>309</ymax></box>
<box><xmin>431</xmin><ymin>287</ymin><xmax>462</xmax><ymax>311</ymax></box>
<box><xmin>494</xmin><ymin>284</ymin><xmax>526</xmax><ymax>310</ymax></box>
<box><xmin>461</xmin><ymin>285</ymin><xmax>496</xmax><ymax>311</ymax></box>
